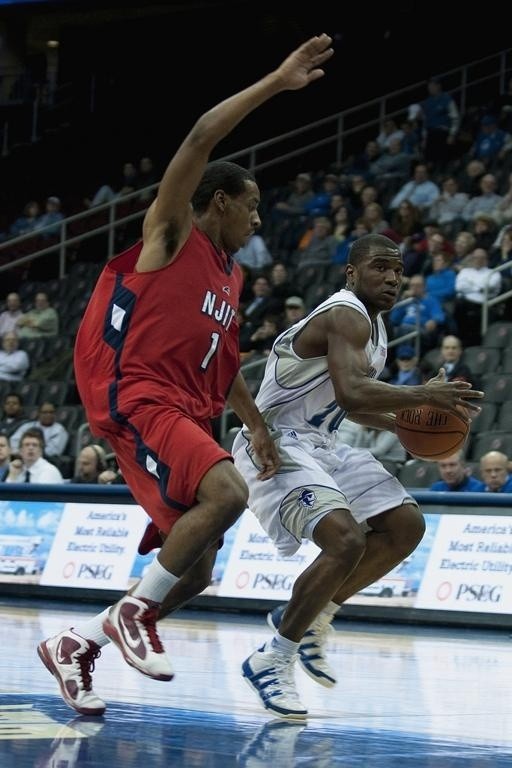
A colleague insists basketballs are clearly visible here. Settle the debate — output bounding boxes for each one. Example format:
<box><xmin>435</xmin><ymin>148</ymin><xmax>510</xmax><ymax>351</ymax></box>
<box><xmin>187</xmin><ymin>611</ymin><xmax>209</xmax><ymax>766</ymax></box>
<box><xmin>394</xmin><ymin>399</ymin><xmax>470</xmax><ymax>461</ymax></box>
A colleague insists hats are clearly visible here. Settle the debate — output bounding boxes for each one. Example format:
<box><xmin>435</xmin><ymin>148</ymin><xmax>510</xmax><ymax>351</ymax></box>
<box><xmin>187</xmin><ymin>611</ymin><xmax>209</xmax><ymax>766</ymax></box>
<box><xmin>397</xmin><ymin>345</ymin><xmax>415</xmax><ymax>358</ymax></box>
<box><xmin>285</xmin><ymin>295</ymin><xmax>304</xmax><ymax>308</ymax></box>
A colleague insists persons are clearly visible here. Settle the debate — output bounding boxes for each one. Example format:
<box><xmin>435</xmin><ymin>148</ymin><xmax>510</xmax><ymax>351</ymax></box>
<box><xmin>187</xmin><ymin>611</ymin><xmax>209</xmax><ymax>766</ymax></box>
<box><xmin>37</xmin><ymin>715</ymin><xmax>107</xmax><ymax>768</ymax></box>
<box><xmin>335</xmin><ymin>336</ymin><xmax>511</xmax><ymax>506</ymax></box>
<box><xmin>37</xmin><ymin>34</ymin><xmax>333</xmax><ymax>717</ymax></box>
<box><xmin>233</xmin><ymin>234</ymin><xmax>487</xmax><ymax>723</ymax></box>
<box><xmin>236</xmin><ymin>715</ymin><xmax>335</xmax><ymax>768</ymax></box>
<box><xmin>0</xmin><ymin>160</ymin><xmax>157</xmax><ymax>485</ymax></box>
<box><xmin>232</xmin><ymin>74</ymin><xmax>510</xmax><ymax>364</ymax></box>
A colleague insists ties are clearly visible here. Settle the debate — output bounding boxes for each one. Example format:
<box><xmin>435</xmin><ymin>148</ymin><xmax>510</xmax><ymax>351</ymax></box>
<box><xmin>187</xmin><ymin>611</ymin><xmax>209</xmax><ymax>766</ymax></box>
<box><xmin>25</xmin><ymin>470</ymin><xmax>29</xmax><ymax>481</ymax></box>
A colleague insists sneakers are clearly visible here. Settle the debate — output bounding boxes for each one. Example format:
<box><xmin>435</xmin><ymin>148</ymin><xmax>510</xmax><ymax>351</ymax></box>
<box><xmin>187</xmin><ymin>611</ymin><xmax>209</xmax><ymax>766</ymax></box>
<box><xmin>36</xmin><ymin>626</ymin><xmax>106</xmax><ymax>714</ymax></box>
<box><xmin>242</xmin><ymin>640</ymin><xmax>309</xmax><ymax>718</ymax></box>
<box><xmin>266</xmin><ymin>602</ymin><xmax>337</xmax><ymax>687</ymax></box>
<box><xmin>102</xmin><ymin>594</ymin><xmax>175</xmax><ymax>680</ymax></box>
